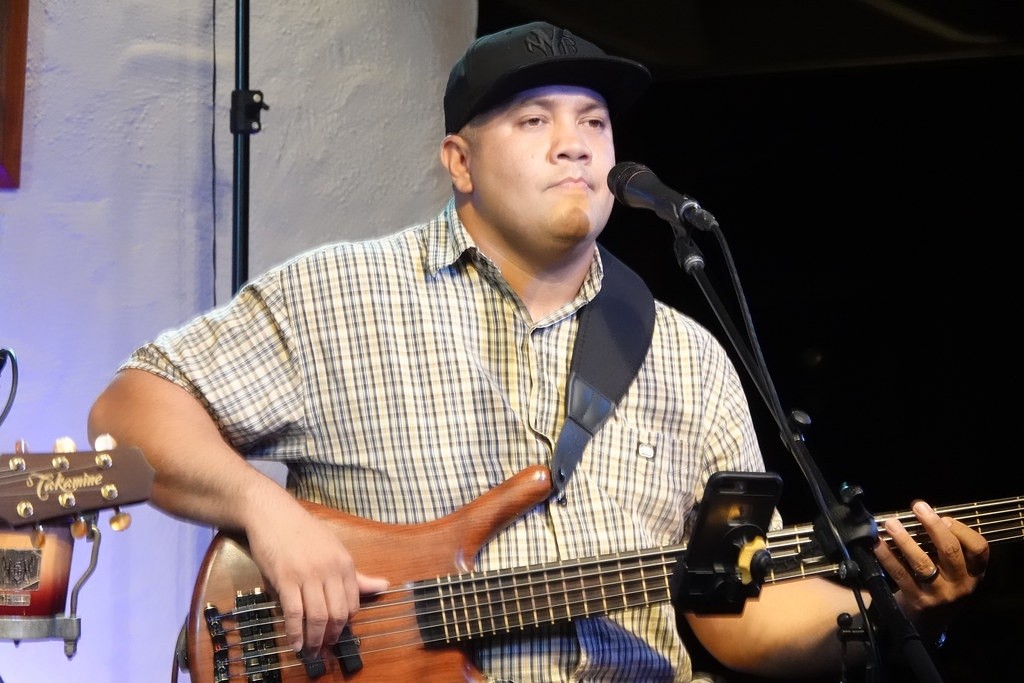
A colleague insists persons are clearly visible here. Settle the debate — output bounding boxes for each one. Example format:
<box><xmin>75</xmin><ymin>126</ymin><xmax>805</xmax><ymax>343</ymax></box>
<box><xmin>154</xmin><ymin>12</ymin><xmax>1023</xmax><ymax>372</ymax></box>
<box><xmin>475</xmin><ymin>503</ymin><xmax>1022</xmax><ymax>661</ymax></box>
<box><xmin>89</xmin><ymin>22</ymin><xmax>988</xmax><ymax>683</ymax></box>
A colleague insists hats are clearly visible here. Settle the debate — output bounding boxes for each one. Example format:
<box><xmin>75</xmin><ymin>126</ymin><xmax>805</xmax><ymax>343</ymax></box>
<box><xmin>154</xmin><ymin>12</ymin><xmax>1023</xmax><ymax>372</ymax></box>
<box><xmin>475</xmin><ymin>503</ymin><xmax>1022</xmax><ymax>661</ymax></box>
<box><xmin>441</xmin><ymin>21</ymin><xmax>650</xmax><ymax>134</ymax></box>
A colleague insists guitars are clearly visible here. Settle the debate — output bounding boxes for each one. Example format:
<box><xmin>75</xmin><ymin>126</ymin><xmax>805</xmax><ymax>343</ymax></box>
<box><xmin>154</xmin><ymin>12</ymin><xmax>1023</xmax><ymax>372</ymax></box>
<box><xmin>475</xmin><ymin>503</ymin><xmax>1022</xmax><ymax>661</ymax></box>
<box><xmin>0</xmin><ymin>432</ymin><xmax>159</xmax><ymax>545</ymax></box>
<box><xmin>185</xmin><ymin>463</ymin><xmax>1024</xmax><ymax>683</ymax></box>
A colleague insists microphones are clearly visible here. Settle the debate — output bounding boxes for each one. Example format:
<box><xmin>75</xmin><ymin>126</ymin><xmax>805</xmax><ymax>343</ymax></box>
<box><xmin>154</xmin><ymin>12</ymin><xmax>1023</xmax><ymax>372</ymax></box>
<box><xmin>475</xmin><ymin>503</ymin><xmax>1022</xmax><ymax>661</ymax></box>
<box><xmin>607</xmin><ymin>161</ymin><xmax>719</xmax><ymax>231</ymax></box>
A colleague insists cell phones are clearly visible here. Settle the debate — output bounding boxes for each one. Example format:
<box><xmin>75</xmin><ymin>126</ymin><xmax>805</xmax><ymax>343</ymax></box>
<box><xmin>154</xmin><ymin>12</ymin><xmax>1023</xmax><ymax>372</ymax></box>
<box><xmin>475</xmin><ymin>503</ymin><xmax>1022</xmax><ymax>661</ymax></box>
<box><xmin>670</xmin><ymin>471</ymin><xmax>784</xmax><ymax>616</ymax></box>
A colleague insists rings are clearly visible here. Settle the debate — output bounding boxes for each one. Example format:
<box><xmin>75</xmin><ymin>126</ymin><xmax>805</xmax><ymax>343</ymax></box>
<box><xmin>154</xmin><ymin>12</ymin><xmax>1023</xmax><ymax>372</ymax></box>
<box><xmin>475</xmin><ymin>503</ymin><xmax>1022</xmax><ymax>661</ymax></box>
<box><xmin>917</xmin><ymin>564</ymin><xmax>940</xmax><ymax>583</ymax></box>
